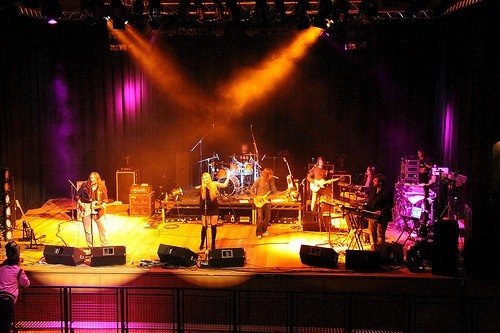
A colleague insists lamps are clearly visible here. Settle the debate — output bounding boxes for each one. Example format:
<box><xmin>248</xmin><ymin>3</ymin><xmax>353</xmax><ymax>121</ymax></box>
<box><xmin>41</xmin><ymin>0</ymin><xmax>380</xmax><ymax>51</ymax></box>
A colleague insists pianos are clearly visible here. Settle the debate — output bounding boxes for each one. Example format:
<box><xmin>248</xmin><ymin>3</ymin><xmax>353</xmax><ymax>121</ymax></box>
<box><xmin>339</xmin><ymin>205</ymin><xmax>381</xmax><ymax>250</ymax></box>
<box><xmin>342</xmin><ymin>193</ymin><xmax>370</xmax><ymax>203</ymax></box>
<box><xmin>338</xmin><ymin>181</ymin><xmax>370</xmax><ymax>191</ymax></box>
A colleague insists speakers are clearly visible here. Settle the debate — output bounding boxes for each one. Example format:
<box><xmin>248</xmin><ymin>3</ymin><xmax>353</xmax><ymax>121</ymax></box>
<box><xmin>300</xmin><ymin>244</ymin><xmax>338</xmax><ymax>268</ymax></box>
<box><xmin>345</xmin><ymin>250</ymin><xmax>380</xmax><ymax>271</ymax></box>
<box><xmin>208</xmin><ymin>247</ymin><xmax>245</xmax><ymax>267</ymax></box>
<box><xmin>130</xmin><ymin>191</ymin><xmax>155</xmax><ymax>218</ymax></box>
<box><xmin>431</xmin><ymin>221</ymin><xmax>458</xmax><ymax>275</ymax></box>
<box><xmin>302</xmin><ymin>215</ymin><xmax>331</xmax><ymax>232</ymax></box>
<box><xmin>90</xmin><ymin>245</ymin><xmax>126</xmax><ymax>267</ymax></box>
<box><xmin>43</xmin><ymin>244</ymin><xmax>86</xmax><ymax>267</ymax></box>
<box><xmin>158</xmin><ymin>244</ymin><xmax>198</xmax><ymax>267</ymax></box>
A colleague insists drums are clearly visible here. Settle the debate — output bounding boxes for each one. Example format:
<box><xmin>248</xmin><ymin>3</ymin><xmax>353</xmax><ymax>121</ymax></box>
<box><xmin>217</xmin><ymin>169</ymin><xmax>229</xmax><ymax>182</ymax></box>
<box><xmin>233</xmin><ymin>170</ymin><xmax>254</xmax><ymax>188</ymax></box>
<box><xmin>217</xmin><ymin>177</ymin><xmax>240</xmax><ymax>194</ymax></box>
<box><xmin>229</xmin><ymin>163</ymin><xmax>239</xmax><ymax>173</ymax></box>
<box><xmin>244</xmin><ymin>162</ymin><xmax>253</xmax><ymax>171</ymax></box>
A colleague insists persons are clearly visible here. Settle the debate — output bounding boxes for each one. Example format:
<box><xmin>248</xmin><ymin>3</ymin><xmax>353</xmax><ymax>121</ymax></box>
<box><xmin>199</xmin><ymin>170</ymin><xmax>229</xmax><ymax>251</ymax></box>
<box><xmin>77</xmin><ymin>172</ymin><xmax>108</xmax><ymax>247</ymax></box>
<box><xmin>233</xmin><ymin>141</ymin><xmax>254</xmax><ymax>164</ymax></box>
<box><xmin>361</xmin><ymin>174</ymin><xmax>394</xmax><ymax>251</ymax></box>
<box><xmin>417</xmin><ymin>149</ymin><xmax>440</xmax><ymax>199</ymax></box>
<box><xmin>307</xmin><ymin>156</ymin><xmax>327</xmax><ymax>212</ymax></box>
<box><xmin>250</xmin><ymin>168</ymin><xmax>277</xmax><ymax>239</ymax></box>
<box><xmin>0</xmin><ymin>254</ymin><xmax>30</xmax><ymax>333</ymax></box>
<box><xmin>361</xmin><ymin>166</ymin><xmax>376</xmax><ymax>187</ymax></box>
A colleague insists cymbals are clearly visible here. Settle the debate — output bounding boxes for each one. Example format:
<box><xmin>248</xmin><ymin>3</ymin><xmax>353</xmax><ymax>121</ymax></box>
<box><xmin>243</xmin><ymin>154</ymin><xmax>258</xmax><ymax>155</ymax></box>
<box><xmin>229</xmin><ymin>156</ymin><xmax>239</xmax><ymax>157</ymax></box>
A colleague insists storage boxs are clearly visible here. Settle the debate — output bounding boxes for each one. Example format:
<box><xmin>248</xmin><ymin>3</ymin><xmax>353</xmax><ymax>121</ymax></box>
<box><xmin>400</xmin><ymin>158</ymin><xmax>420</xmax><ymax>183</ymax></box>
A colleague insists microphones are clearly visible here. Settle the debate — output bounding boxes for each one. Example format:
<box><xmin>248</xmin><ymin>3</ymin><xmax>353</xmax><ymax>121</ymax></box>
<box><xmin>262</xmin><ymin>154</ymin><xmax>265</xmax><ymax>160</ymax></box>
<box><xmin>216</xmin><ymin>153</ymin><xmax>219</xmax><ymax>160</ymax></box>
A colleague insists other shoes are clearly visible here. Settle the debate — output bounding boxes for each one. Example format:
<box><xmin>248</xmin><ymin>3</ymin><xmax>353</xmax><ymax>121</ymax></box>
<box><xmin>257</xmin><ymin>233</ymin><xmax>269</xmax><ymax>239</ymax></box>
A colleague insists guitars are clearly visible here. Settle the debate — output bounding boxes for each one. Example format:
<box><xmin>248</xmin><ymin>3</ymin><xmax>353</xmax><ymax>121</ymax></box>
<box><xmin>253</xmin><ymin>187</ymin><xmax>296</xmax><ymax>208</ymax></box>
<box><xmin>310</xmin><ymin>179</ymin><xmax>340</xmax><ymax>193</ymax></box>
<box><xmin>78</xmin><ymin>200</ymin><xmax>122</xmax><ymax>218</ymax></box>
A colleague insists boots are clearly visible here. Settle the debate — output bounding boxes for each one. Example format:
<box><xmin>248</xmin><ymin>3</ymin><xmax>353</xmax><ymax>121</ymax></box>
<box><xmin>200</xmin><ymin>226</ymin><xmax>208</xmax><ymax>250</ymax></box>
<box><xmin>211</xmin><ymin>224</ymin><xmax>217</xmax><ymax>251</ymax></box>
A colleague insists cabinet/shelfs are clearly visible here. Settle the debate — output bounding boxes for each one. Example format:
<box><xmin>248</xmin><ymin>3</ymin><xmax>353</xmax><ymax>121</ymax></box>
<box><xmin>129</xmin><ymin>190</ymin><xmax>156</xmax><ymax>217</ymax></box>
